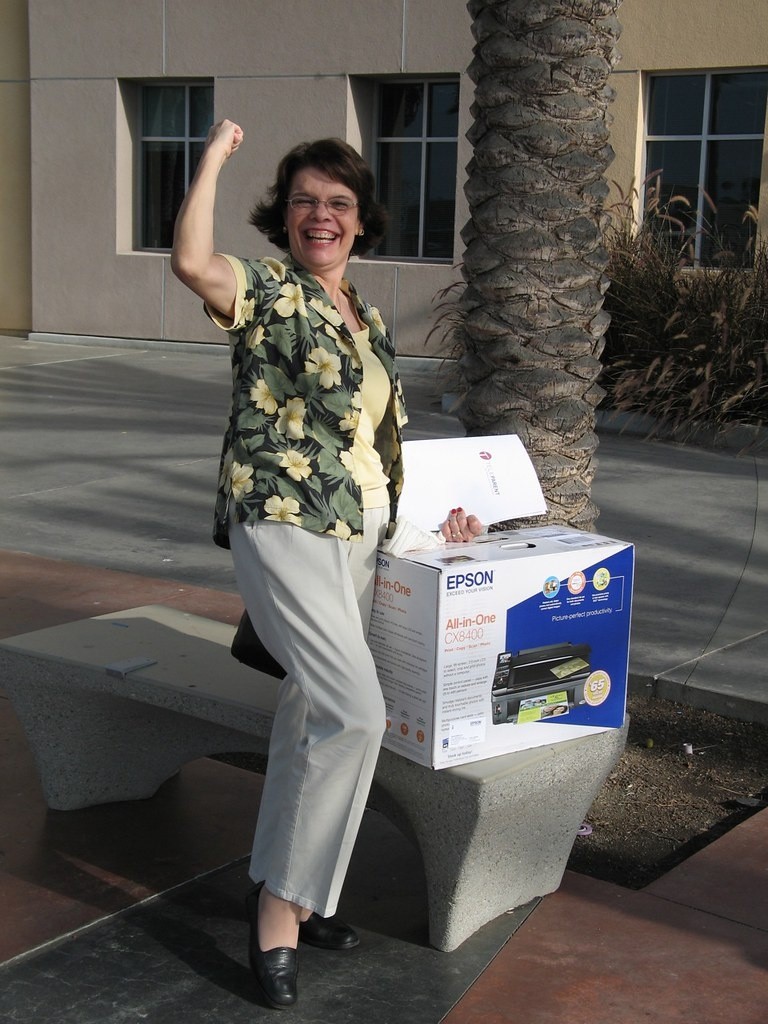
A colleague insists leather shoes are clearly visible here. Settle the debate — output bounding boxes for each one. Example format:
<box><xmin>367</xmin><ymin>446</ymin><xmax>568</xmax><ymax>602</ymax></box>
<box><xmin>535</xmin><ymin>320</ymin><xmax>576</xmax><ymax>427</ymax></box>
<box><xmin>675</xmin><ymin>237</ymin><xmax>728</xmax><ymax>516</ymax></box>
<box><xmin>299</xmin><ymin>913</ymin><xmax>360</xmax><ymax>948</ymax></box>
<box><xmin>245</xmin><ymin>880</ymin><xmax>301</xmax><ymax>1009</ymax></box>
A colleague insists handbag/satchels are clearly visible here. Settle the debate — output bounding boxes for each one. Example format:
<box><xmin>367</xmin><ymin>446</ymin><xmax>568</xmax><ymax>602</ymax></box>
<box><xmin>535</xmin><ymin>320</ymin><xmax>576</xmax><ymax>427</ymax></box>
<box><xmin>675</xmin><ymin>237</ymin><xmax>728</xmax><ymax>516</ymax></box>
<box><xmin>231</xmin><ymin>611</ymin><xmax>288</xmax><ymax>680</ymax></box>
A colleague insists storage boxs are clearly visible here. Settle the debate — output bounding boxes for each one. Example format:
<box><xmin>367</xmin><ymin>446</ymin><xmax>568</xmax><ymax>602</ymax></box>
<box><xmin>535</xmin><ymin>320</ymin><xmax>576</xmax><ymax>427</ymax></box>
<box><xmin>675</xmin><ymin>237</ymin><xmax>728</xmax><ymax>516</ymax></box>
<box><xmin>363</xmin><ymin>526</ymin><xmax>636</xmax><ymax>771</ymax></box>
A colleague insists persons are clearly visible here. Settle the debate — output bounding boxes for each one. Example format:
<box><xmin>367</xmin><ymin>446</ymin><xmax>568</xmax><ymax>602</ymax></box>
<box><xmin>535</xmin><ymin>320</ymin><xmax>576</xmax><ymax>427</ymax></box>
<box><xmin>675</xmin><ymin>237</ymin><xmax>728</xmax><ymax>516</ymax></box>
<box><xmin>541</xmin><ymin>704</ymin><xmax>568</xmax><ymax>717</ymax></box>
<box><xmin>170</xmin><ymin>118</ymin><xmax>484</xmax><ymax>1010</ymax></box>
<box><xmin>522</xmin><ymin>699</ymin><xmax>544</xmax><ymax>709</ymax></box>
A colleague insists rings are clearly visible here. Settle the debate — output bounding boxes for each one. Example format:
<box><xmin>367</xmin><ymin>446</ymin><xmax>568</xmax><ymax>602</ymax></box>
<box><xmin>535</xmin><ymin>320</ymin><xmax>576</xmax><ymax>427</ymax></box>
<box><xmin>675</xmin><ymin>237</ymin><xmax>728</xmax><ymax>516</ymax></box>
<box><xmin>451</xmin><ymin>532</ymin><xmax>461</xmax><ymax>537</ymax></box>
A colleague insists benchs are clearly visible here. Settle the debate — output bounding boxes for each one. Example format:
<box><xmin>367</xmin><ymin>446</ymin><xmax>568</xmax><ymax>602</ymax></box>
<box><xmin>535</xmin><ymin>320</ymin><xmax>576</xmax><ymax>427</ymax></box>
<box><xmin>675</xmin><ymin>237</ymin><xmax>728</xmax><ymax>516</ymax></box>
<box><xmin>0</xmin><ymin>603</ymin><xmax>630</xmax><ymax>953</ymax></box>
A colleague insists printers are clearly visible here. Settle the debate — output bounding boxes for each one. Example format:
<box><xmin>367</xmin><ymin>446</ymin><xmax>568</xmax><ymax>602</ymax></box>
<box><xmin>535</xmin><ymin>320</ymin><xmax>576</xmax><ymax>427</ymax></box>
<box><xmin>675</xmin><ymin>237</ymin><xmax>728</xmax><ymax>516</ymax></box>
<box><xmin>491</xmin><ymin>641</ymin><xmax>592</xmax><ymax>727</ymax></box>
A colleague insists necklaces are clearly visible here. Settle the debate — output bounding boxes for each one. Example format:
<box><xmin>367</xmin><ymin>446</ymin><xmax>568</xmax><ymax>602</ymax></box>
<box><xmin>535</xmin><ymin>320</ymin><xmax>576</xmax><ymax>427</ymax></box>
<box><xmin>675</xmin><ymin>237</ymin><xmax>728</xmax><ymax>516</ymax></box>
<box><xmin>336</xmin><ymin>293</ymin><xmax>342</xmax><ymax>314</ymax></box>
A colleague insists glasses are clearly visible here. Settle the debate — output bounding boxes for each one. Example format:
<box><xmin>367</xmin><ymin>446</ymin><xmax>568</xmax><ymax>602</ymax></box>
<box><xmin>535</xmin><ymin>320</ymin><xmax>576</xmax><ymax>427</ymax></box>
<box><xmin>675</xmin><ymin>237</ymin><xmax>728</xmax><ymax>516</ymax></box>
<box><xmin>286</xmin><ymin>192</ymin><xmax>359</xmax><ymax>213</ymax></box>
